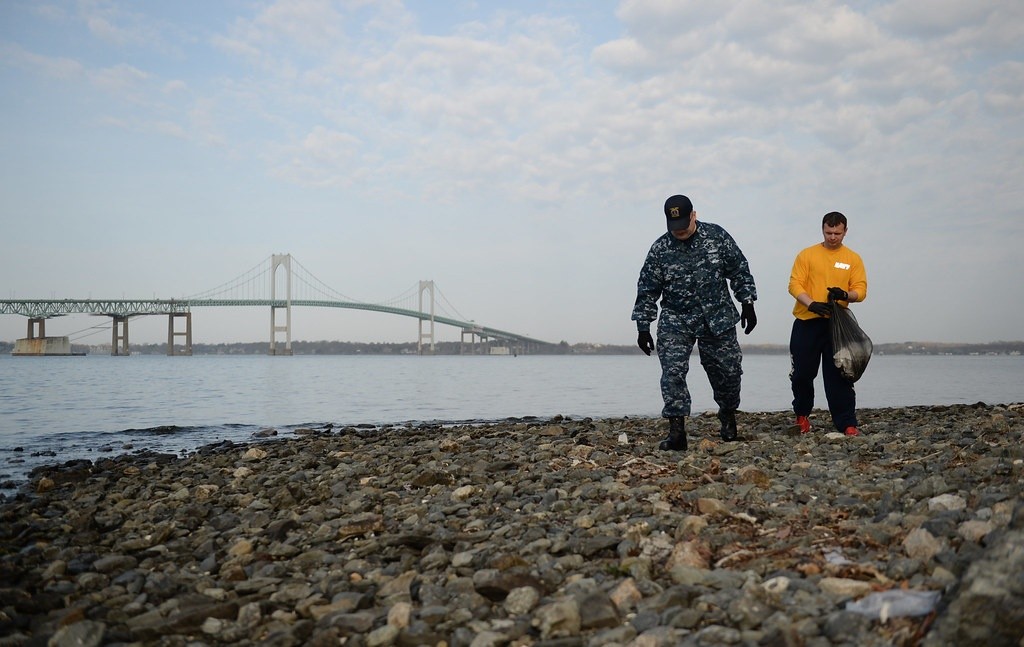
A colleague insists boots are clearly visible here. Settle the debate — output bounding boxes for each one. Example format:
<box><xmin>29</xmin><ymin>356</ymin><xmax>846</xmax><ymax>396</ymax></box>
<box><xmin>658</xmin><ymin>415</ymin><xmax>687</xmax><ymax>451</ymax></box>
<box><xmin>716</xmin><ymin>408</ymin><xmax>736</xmax><ymax>442</ymax></box>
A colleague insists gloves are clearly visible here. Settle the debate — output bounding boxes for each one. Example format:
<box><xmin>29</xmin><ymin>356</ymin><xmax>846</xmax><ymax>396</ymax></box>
<box><xmin>827</xmin><ymin>287</ymin><xmax>849</xmax><ymax>301</ymax></box>
<box><xmin>740</xmin><ymin>299</ymin><xmax>757</xmax><ymax>334</ymax></box>
<box><xmin>808</xmin><ymin>301</ymin><xmax>831</xmax><ymax>316</ymax></box>
<box><xmin>637</xmin><ymin>330</ymin><xmax>654</xmax><ymax>356</ymax></box>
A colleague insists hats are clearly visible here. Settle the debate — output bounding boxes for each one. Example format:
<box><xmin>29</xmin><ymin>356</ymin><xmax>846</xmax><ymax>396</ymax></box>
<box><xmin>663</xmin><ymin>194</ymin><xmax>693</xmax><ymax>230</ymax></box>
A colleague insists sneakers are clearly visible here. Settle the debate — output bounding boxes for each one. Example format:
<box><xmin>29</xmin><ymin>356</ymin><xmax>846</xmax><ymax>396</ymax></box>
<box><xmin>846</xmin><ymin>426</ymin><xmax>860</xmax><ymax>437</ymax></box>
<box><xmin>796</xmin><ymin>416</ymin><xmax>810</xmax><ymax>432</ymax></box>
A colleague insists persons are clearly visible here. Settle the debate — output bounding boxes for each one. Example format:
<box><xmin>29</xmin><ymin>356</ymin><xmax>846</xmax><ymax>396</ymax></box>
<box><xmin>789</xmin><ymin>212</ymin><xmax>867</xmax><ymax>438</ymax></box>
<box><xmin>632</xmin><ymin>194</ymin><xmax>757</xmax><ymax>448</ymax></box>
